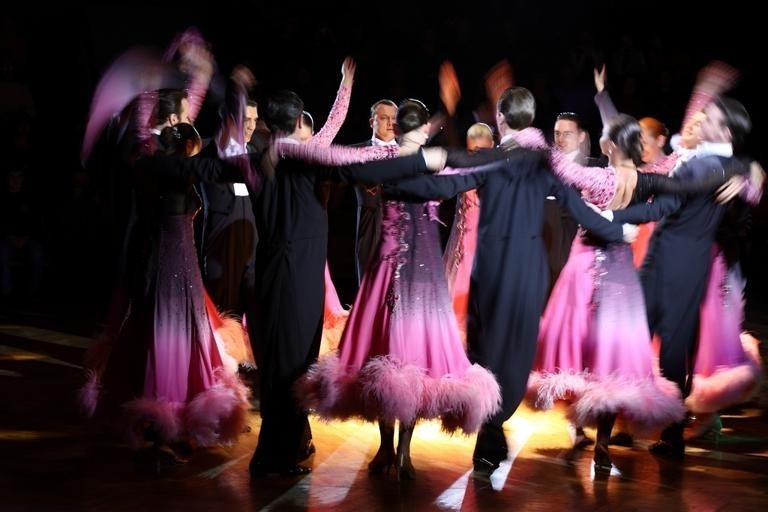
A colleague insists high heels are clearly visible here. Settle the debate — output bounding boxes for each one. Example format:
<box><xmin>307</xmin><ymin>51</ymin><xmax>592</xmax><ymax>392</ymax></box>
<box><xmin>130</xmin><ymin>441</ymin><xmax>191</xmax><ymax>472</ymax></box>
<box><xmin>683</xmin><ymin>414</ymin><xmax>722</xmax><ymax>444</ymax></box>
<box><xmin>367</xmin><ymin>445</ymin><xmax>397</xmax><ymax>475</ymax></box>
<box><xmin>394</xmin><ymin>451</ymin><xmax>416</xmax><ymax>482</ymax></box>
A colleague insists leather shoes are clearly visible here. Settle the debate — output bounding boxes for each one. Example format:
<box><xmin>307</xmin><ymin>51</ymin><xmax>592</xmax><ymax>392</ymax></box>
<box><xmin>648</xmin><ymin>440</ymin><xmax>685</xmax><ymax>459</ymax></box>
<box><xmin>473</xmin><ymin>441</ymin><xmax>507</xmax><ymax>483</ymax></box>
<box><xmin>566</xmin><ymin>437</ymin><xmax>593</xmax><ymax>457</ymax></box>
<box><xmin>608</xmin><ymin>433</ymin><xmax>632</xmax><ymax>447</ymax></box>
<box><xmin>249</xmin><ymin>440</ymin><xmax>316</xmax><ymax>480</ymax></box>
<box><xmin>595</xmin><ymin>442</ymin><xmax>611</xmax><ymax>476</ymax></box>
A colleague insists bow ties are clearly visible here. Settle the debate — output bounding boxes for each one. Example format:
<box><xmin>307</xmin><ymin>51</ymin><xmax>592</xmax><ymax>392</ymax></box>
<box><xmin>377</xmin><ymin>140</ymin><xmax>396</xmax><ymax>146</ymax></box>
